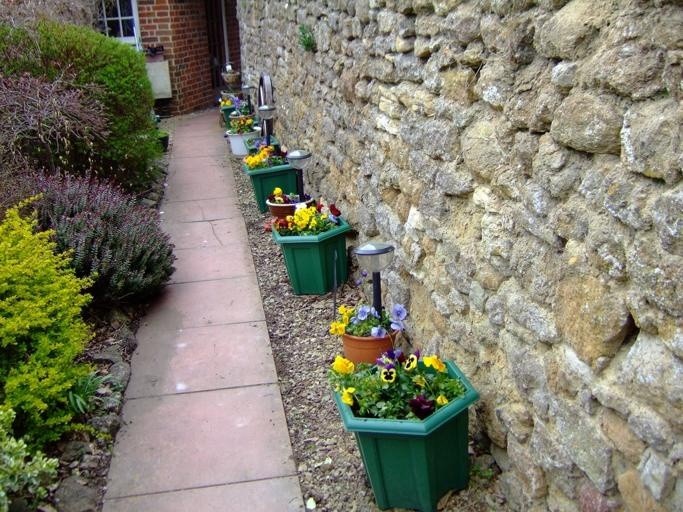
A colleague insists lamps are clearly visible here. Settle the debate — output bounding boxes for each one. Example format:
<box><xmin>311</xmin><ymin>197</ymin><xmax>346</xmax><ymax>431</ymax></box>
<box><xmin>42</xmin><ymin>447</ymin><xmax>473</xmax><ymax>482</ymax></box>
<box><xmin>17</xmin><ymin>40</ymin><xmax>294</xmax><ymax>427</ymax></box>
<box><xmin>354</xmin><ymin>241</ymin><xmax>396</xmax><ymax>323</ymax></box>
<box><xmin>258</xmin><ymin>104</ymin><xmax>278</xmax><ymax>145</ymax></box>
<box><xmin>286</xmin><ymin>148</ymin><xmax>312</xmax><ymax>200</ymax></box>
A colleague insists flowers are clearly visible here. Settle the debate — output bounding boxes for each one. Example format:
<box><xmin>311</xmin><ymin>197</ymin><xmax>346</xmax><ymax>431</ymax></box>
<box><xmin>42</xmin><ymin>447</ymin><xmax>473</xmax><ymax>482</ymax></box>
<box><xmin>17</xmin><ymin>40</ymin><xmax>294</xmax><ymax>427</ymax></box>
<box><xmin>241</xmin><ymin>136</ymin><xmax>285</xmax><ymax>167</ymax></box>
<box><xmin>330</xmin><ymin>347</ymin><xmax>466</xmax><ymax>422</ymax></box>
<box><xmin>265</xmin><ymin>185</ymin><xmax>342</xmax><ymax>236</ymax></box>
<box><xmin>217</xmin><ymin>93</ymin><xmax>257</xmax><ymax>133</ymax></box>
<box><xmin>326</xmin><ymin>300</ymin><xmax>409</xmax><ymax>345</ymax></box>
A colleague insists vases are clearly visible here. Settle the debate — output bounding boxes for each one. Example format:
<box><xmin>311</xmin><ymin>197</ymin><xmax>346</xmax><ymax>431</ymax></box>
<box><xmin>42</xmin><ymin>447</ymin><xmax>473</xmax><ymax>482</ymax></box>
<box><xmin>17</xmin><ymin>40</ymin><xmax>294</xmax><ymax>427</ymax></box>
<box><xmin>341</xmin><ymin>332</ymin><xmax>400</xmax><ymax>371</ymax></box>
<box><xmin>271</xmin><ymin>218</ymin><xmax>353</xmax><ymax>296</ymax></box>
<box><xmin>226</xmin><ymin>127</ymin><xmax>262</xmax><ymax>156</ymax></box>
<box><xmin>243</xmin><ymin>164</ymin><xmax>299</xmax><ymax>215</ymax></box>
<box><xmin>330</xmin><ymin>358</ymin><xmax>480</xmax><ymax>511</ymax></box>
